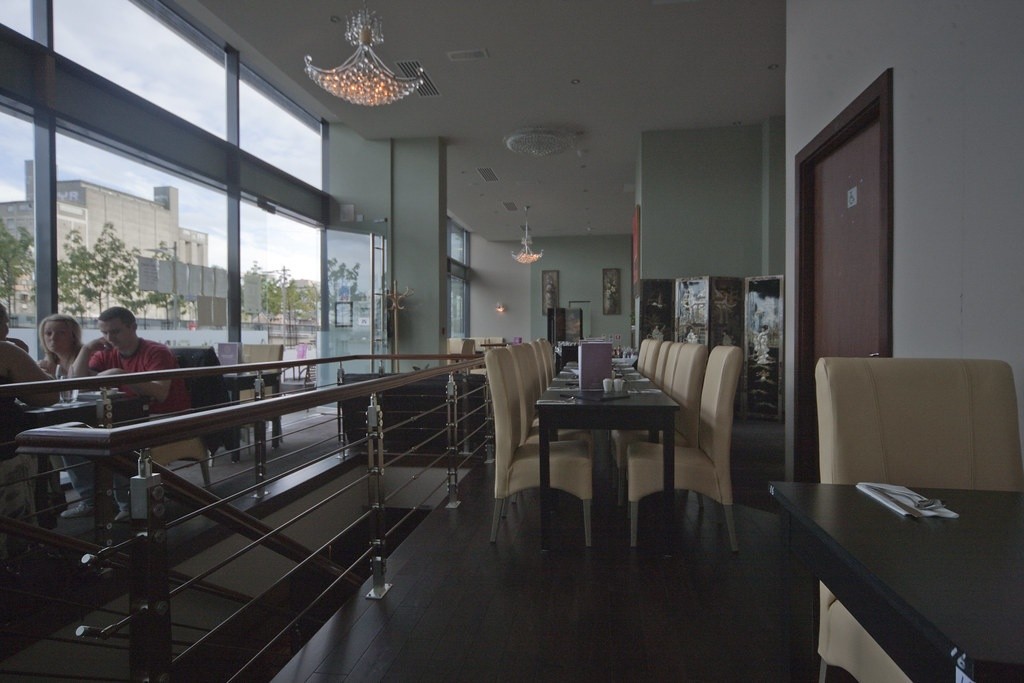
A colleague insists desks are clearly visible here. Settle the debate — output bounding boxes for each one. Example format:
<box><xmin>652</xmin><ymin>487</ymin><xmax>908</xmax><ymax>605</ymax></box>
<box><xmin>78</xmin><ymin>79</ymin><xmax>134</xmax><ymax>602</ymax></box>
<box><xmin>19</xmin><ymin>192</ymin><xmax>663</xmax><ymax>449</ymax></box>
<box><xmin>221</xmin><ymin>372</ymin><xmax>282</xmax><ymax>462</ymax></box>
<box><xmin>19</xmin><ymin>395</ymin><xmax>150</xmax><ymax>429</ymax></box>
<box><xmin>536</xmin><ymin>361</ymin><xmax>682</xmax><ymax>549</ymax></box>
<box><xmin>764</xmin><ymin>478</ymin><xmax>1024</xmax><ymax>683</ymax></box>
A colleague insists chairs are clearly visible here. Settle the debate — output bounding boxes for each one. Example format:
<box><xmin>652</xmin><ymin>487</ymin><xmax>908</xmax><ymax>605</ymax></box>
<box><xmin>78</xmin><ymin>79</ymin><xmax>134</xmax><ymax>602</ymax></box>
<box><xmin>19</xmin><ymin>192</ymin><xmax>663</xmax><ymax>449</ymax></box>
<box><xmin>147</xmin><ymin>342</ymin><xmax>291</xmax><ymax>489</ymax></box>
<box><xmin>812</xmin><ymin>352</ymin><xmax>1024</xmax><ymax>683</ymax></box>
<box><xmin>480</xmin><ymin>336</ymin><xmax>593</xmax><ymax>548</ymax></box>
<box><xmin>609</xmin><ymin>338</ymin><xmax>743</xmax><ymax>552</ymax></box>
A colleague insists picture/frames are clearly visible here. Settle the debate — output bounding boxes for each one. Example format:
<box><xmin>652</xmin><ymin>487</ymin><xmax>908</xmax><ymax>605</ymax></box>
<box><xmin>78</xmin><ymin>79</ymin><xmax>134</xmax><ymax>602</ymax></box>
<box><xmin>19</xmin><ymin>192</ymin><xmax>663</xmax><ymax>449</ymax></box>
<box><xmin>602</xmin><ymin>268</ymin><xmax>622</xmax><ymax>315</ymax></box>
<box><xmin>542</xmin><ymin>269</ymin><xmax>559</xmax><ymax>316</ymax></box>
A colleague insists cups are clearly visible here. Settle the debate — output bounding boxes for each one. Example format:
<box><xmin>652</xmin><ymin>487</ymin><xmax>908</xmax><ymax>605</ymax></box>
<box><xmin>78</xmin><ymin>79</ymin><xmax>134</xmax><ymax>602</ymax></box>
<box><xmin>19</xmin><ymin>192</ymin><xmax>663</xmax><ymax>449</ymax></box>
<box><xmin>55</xmin><ymin>364</ymin><xmax>76</xmax><ymax>380</ymax></box>
<box><xmin>614</xmin><ymin>379</ymin><xmax>624</xmax><ymax>392</ymax></box>
<box><xmin>603</xmin><ymin>378</ymin><xmax>613</xmax><ymax>392</ymax></box>
<box><xmin>60</xmin><ymin>376</ymin><xmax>79</xmax><ymax>403</ymax></box>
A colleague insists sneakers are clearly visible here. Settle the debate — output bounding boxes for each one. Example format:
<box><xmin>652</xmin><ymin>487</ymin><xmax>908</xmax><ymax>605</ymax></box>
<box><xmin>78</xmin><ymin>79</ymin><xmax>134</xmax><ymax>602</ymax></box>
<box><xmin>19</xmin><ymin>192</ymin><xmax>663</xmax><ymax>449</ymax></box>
<box><xmin>114</xmin><ymin>509</ymin><xmax>131</xmax><ymax>522</ymax></box>
<box><xmin>60</xmin><ymin>501</ymin><xmax>95</xmax><ymax>518</ymax></box>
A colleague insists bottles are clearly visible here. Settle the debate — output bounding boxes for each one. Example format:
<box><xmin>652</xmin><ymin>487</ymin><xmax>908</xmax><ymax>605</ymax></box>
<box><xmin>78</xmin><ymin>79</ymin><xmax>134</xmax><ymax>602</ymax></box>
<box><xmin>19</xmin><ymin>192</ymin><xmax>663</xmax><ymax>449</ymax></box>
<box><xmin>613</xmin><ymin>345</ymin><xmax>633</xmax><ymax>358</ymax></box>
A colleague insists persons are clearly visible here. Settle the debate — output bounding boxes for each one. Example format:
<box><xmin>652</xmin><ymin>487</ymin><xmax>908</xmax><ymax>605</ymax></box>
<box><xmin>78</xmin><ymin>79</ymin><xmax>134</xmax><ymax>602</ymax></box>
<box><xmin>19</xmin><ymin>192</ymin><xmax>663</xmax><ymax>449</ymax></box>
<box><xmin>36</xmin><ymin>314</ymin><xmax>86</xmax><ymax>402</ymax></box>
<box><xmin>60</xmin><ymin>306</ymin><xmax>195</xmax><ymax>520</ymax></box>
<box><xmin>0</xmin><ymin>303</ymin><xmax>69</xmax><ymax>514</ymax></box>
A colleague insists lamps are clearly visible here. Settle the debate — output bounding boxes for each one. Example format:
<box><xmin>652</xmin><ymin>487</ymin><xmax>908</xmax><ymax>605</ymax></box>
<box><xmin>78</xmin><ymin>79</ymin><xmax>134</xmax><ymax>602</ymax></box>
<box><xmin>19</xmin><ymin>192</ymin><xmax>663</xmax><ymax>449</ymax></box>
<box><xmin>303</xmin><ymin>0</ymin><xmax>425</xmax><ymax>106</ymax></box>
<box><xmin>511</xmin><ymin>208</ymin><xmax>544</xmax><ymax>262</ymax></box>
